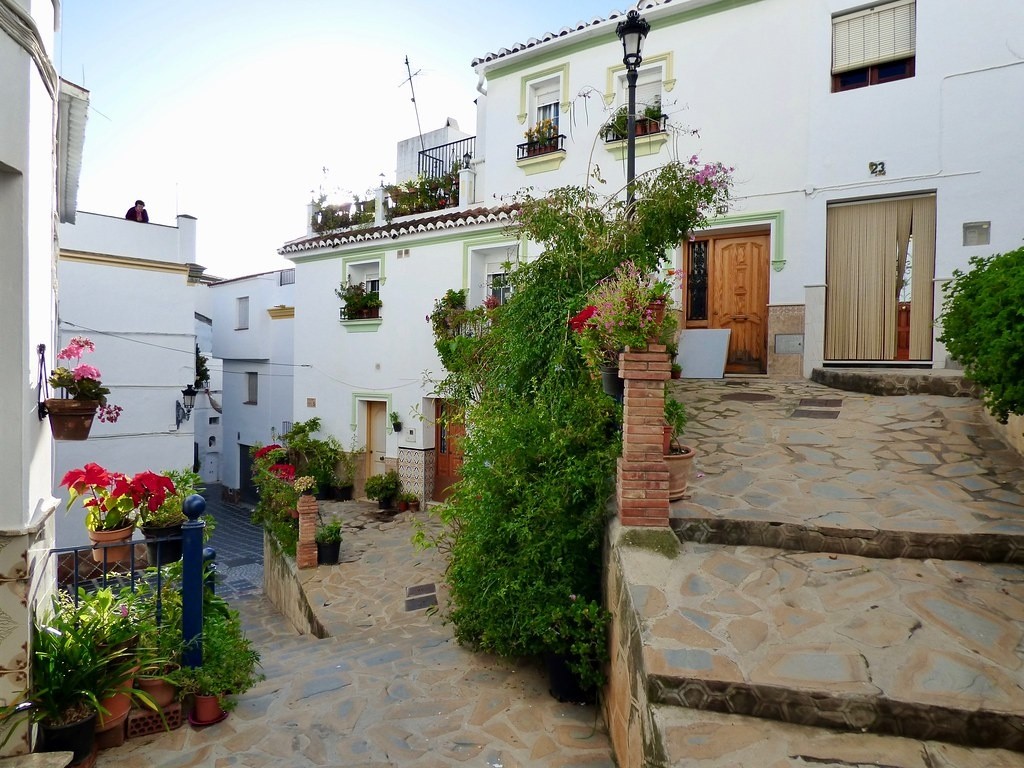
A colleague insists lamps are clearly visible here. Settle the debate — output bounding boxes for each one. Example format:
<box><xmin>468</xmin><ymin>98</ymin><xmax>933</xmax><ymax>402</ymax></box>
<box><xmin>463</xmin><ymin>153</ymin><xmax>471</xmax><ymax>170</ymax></box>
<box><xmin>176</xmin><ymin>385</ymin><xmax>198</xmax><ymax>429</ymax></box>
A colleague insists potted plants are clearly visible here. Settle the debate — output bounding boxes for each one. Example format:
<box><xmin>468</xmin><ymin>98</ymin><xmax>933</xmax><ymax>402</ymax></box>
<box><xmin>389</xmin><ymin>412</ymin><xmax>402</xmax><ymax>432</ymax></box>
<box><xmin>365</xmin><ymin>468</ymin><xmax>420</xmax><ymax>513</ymax></box>
<box><xmin>0</xmin><ymin>568</ymin><xmax>265</xmax><ymax>760</ymax></box>
<box><xmin>612</xmin><ymin>105</ymin><xmax>661</xmax><ymax>140</ymax></box>
<box><xmin>333</xmin><ymin>274</ymin><xmax>383</xmax><ymax>320</ymax></box>
<box><xmin>661</xmin><ymin>400</ymin><xmax>696</xmax><ymax>501</ymax></box>
<box><xmin>250</xmin><ymin>418</ymin><xmax>364</xmax><ymax>565</ymax></box>
<box><xmin>379</xmin><ymin>160</ymin><xmax>460</xmax><ymax>219</ymax></box>
<box><xmin>142</xmin><ymin>469</ymin><xmax>217</xmax><ymax>567</ymax></box>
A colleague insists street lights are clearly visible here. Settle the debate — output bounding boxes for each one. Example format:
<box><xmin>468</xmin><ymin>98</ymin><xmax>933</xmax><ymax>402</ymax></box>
<box><xmin>616</xmin><ymin>9</ymin><xmax>654</xmax><ymax>232</ymax></box>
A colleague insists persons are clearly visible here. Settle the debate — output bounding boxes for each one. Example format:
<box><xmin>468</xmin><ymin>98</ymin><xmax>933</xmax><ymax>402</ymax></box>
<box><xmin>124</xmin><ymin>200</ymin><xmax>149</xmax><ymax>223</ymax></box>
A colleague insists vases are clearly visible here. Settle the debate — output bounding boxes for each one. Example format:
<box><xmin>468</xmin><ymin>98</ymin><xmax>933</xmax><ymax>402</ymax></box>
<box><xmin>302</xmin><ymin>489</ymin><xmax>313</xmax><ymax>495</ymax></box>
<box><xmin>600</xmin><ymin>301</ymin><xmax>665</xmax><ymax>397</ymax></box>
<box><xmin>88</xmin><ymin>519</ymin><xmax>134</xmax><ymax>563</ymax></box>
<box><xmin>527</xmin><ymin>146</ymin><xmax>557</xmax><ymax>157</ymax></box>
<box><xmin>45</xmin><ymin>399</ymin><xmax>98</xmax><ymax>440</ymax></box>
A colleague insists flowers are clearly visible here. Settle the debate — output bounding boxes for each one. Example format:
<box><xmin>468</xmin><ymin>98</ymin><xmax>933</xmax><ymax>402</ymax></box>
<box><xmin>570</xmin><ymin>258</ymin><xmax>685</xmax><ymax>369</ymax></box>
<box><xmin>58</xmin><ymin>460</ymin><xmax>177</xmax><ymax>530</ymax></box>
<box><xmin>522</xmin><ymin>119</ymin><xmax>558</xmax><ymax>147</ymax></box>
<box><xmin>48</xmin><ymin>334</ymin><xmax>123</xmax><ymax>423</ymax></box>
<box><xmin>293</xmin><ymin>475</ymin><xmax>316</xmax><ymax>492</ymax></box>
